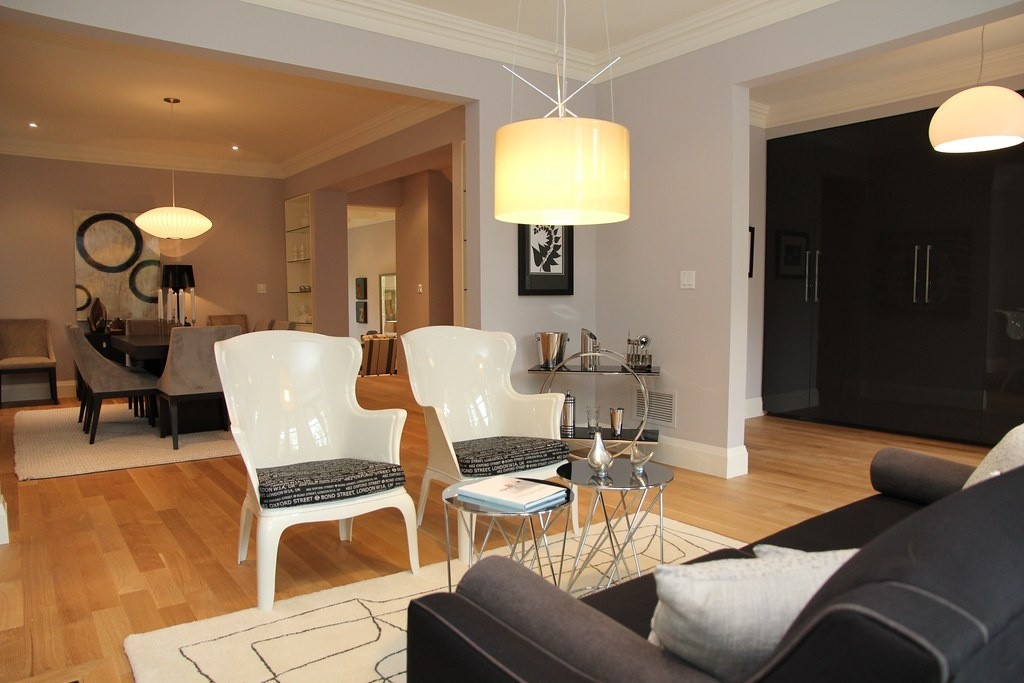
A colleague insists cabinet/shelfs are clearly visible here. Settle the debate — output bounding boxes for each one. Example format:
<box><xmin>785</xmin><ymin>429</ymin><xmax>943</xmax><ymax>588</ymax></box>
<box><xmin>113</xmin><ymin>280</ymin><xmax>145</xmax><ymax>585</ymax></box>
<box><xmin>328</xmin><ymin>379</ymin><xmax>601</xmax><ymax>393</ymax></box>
<box><xmin>527</xmin><ymin>363</ymin><xmax>659</xmax><ymax>445</ymax></box>
<box><xmin>284</xmin><ymin>192</ymin><xmax>314</xmax><ymax>325</ymax></box>
<box><xmin>762</xmin><ymin>88</ymin><xmax>1024</xmax><ymax>445</ymax></box>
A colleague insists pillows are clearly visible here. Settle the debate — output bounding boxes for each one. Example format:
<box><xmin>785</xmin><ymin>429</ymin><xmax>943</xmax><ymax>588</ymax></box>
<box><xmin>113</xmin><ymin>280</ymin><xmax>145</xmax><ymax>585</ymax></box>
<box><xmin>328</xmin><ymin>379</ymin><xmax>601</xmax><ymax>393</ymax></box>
<box><xmin>644</xmin><ymin>544</ymin><xmax>862</xmax><ymax>682</ymax></box>
<box><xmin>963</xmin><ymin>422</ymin><xmax>1024</xmax><ymax>491</ymax></box>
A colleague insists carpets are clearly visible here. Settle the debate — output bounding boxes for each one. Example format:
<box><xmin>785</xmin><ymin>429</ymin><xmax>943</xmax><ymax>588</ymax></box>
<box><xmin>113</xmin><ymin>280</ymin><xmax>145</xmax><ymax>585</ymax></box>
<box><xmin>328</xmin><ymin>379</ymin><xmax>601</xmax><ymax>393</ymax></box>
<box><xmin>13</xmin><ymin>401</ymin><xmax>242</xmax><ymax>482</ymax></box>
<box><xmin>121</xmin><ymin>512</ymin><xmax>749</xmax><ymax>683</ymax></box>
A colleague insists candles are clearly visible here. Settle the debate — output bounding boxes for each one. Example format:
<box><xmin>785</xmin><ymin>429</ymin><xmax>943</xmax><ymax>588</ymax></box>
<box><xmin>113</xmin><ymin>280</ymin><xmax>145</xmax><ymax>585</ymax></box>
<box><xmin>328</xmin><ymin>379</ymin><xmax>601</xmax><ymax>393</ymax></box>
<box><xmin>158</xmin><ymin>287</ymin><xmax>196</xmax><ymax>326</ymax></box>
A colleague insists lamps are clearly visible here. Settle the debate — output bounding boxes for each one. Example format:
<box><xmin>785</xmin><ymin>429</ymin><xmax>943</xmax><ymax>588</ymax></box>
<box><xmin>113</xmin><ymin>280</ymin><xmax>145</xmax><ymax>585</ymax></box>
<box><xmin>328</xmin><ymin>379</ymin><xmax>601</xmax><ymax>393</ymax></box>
<box><xmin>136</xmin><ymin>97</ymin><xmax>212</xmax><ymax>240</ymax></box>
<box><xmin>493</xmin><ymin>0</ymin><xmax>631</xmax><ymax>226</ymax></box>
<box><xmin>162</xmin><ymin>265</ymin><xmax>196</xmax><ymax>327</ymax></box>
<box><xmin>928</xmin><ymin>25</ymin><xmax>1024</xmax><ymax>153</ymax></box>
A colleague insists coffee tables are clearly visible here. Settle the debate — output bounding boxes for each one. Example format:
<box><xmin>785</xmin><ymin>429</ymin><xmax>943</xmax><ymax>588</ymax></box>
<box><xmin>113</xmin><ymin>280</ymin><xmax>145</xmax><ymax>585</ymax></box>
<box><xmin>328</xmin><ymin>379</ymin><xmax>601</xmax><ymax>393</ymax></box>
<box><xmin>441</xmin><ymin>476</ymin><xmax>574</xmax><ymax>593</ymax></box>
<box><xmin>556</xmin><ymin>458</ymin><xmax>674</xmax><ymax>595</ymax></box>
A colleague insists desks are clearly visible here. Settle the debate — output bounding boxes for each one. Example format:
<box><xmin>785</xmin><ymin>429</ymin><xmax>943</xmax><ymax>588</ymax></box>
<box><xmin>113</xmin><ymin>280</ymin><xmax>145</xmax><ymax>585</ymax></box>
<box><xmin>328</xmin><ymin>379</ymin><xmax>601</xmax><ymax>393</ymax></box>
<box><xmin>111</xmin><ymin>335</ymin><xmax>170</xmax><ymax>423</ymax></box>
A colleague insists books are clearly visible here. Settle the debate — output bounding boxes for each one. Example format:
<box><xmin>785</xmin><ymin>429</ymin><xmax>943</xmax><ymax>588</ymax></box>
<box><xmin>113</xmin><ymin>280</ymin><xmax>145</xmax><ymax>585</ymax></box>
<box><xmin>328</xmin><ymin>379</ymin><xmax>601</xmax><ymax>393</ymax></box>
<box><xmin>458</xmin><ymin>495</ymin><xmax>567</xmax><ymax>513</ymax></box>
<box><xmin>457</xmin><ymin>475</ymin><xmax>567</xmax><ymax>510</ymax></box>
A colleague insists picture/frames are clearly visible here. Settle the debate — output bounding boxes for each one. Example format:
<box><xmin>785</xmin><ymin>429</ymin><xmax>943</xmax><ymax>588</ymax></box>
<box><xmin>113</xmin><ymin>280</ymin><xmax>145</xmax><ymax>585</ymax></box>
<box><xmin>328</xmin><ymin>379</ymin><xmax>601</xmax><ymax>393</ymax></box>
<box><xmin>518</xmin><ymin>224</ymin><xmax>574</xmax><ymax>296</ymax></box>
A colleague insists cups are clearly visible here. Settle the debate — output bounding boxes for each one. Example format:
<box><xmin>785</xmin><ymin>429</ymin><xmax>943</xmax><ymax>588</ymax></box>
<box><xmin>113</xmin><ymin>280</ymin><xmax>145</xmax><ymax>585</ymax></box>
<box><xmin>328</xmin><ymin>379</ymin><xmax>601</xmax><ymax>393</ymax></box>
<box><xmin>586</xmin><ymin>406</ymin><xmax>600</xmax><ymax>431</ymax></box>
<box><xmin>610</xmin><ymin>408</ymin><xmax>623</xmax><ymax>436</ymax></box>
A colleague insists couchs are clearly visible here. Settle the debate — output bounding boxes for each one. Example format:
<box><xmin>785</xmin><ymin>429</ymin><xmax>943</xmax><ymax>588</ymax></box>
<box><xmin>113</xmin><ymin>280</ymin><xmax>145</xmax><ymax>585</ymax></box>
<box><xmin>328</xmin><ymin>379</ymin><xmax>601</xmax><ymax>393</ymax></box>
<box><xmin>357</xmin><ymin>333</ymin><xmax>397</xmax><ymax>377</ymax></box>
<box><xmin>407</xmin><ymin>449</ymin><xmax>1024</xmax><ymax>683</ymax></box>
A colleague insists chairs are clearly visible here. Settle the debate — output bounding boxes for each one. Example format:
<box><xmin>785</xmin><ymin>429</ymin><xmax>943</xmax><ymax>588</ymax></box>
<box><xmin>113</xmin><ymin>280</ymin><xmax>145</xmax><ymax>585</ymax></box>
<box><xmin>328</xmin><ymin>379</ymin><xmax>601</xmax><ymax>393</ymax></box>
<box><xmin>66</xmin><ymin>314</ymin><xmax>277</xmax><ymax>449</ymax></box>
<box><xmin>214</xmin><ymin>331</ymin><xmax>419</xmax><ymax>613</ymax></box>
<box><xmin>0</xmin><ymin>319</ymin><xmax>59</xmax><ymax>404</ymax></box>
<box><xmin>401</xmin><ymin>326</ymin><xmax>578</xmax><ymax>566</ymax></box>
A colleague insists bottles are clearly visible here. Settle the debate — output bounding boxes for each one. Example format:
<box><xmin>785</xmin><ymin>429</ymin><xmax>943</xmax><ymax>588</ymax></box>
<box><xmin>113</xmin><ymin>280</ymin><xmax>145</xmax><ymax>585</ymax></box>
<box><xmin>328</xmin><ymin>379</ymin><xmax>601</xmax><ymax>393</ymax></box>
<box><xmin>562</xmin><ymin>389</ymin><xmax>576</xmax><ymax>433</ymax></box>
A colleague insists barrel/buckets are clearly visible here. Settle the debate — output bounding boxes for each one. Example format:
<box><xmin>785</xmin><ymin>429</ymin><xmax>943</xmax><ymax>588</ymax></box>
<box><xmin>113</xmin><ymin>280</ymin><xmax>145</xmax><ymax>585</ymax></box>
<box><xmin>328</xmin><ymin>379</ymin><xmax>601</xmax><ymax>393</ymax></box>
<box><xmin>535</xmin><ymin>331</ymin><xmax>568</xmax><ymax>369</ymax></box>
